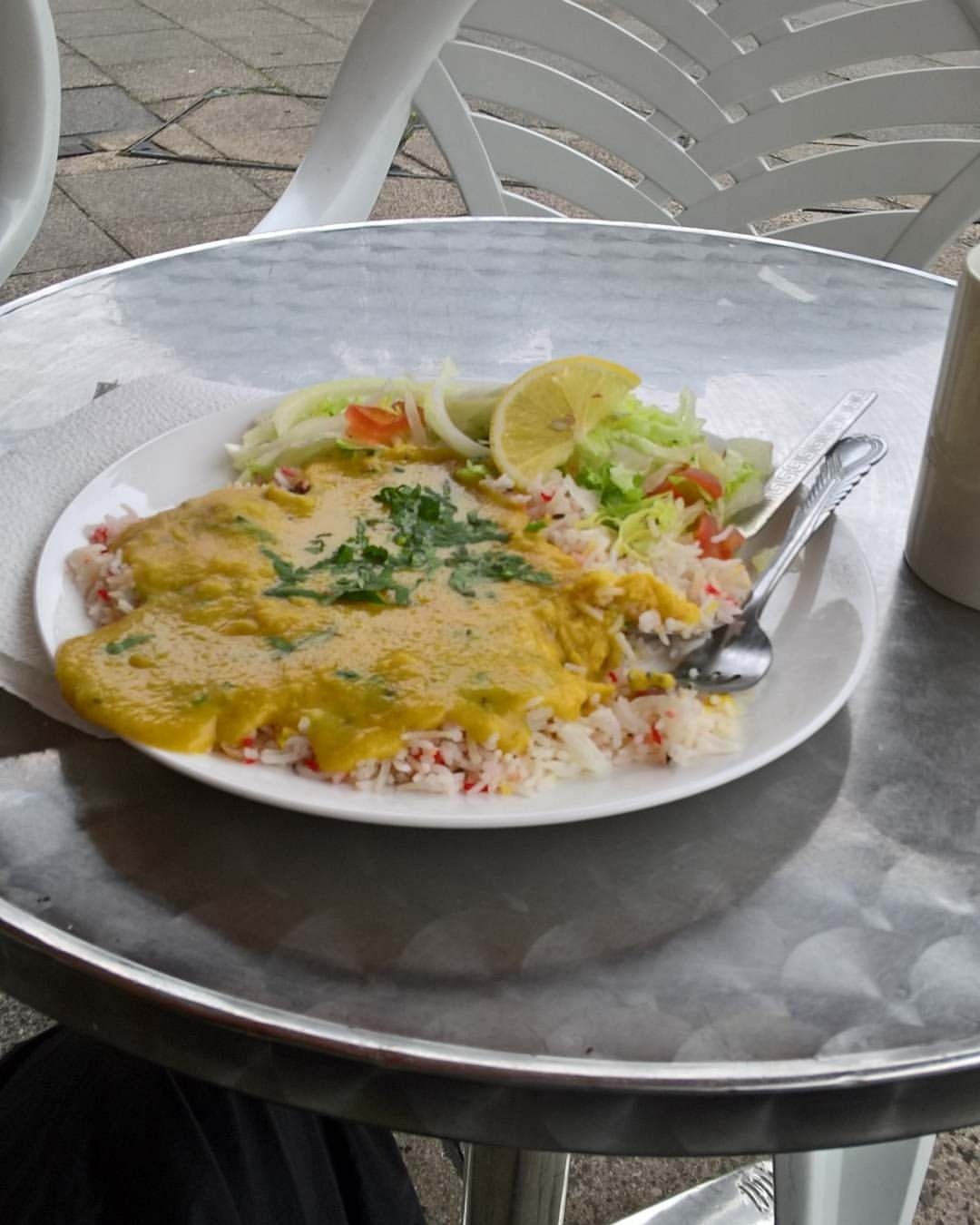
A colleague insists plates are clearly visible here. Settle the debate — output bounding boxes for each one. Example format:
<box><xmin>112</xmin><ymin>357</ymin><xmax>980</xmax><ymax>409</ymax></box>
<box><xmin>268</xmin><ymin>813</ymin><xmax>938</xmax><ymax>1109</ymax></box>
<box><xmin>34</xmin><ymin>377</ymin><xmax>880</xmax><ymax>829</ymax></box>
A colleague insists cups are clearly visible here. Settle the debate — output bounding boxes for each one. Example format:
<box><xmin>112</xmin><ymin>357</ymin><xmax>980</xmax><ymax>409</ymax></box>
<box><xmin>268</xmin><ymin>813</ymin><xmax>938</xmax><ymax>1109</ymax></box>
<box><xmin>905</xmin><ymin>234</ymin><xmax>980</xmax><ymax>615</ymax></box>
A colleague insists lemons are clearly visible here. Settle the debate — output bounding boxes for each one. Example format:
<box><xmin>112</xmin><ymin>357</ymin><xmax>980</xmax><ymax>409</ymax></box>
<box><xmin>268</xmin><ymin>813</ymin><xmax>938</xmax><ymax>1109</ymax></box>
<box><xmin>486</xmin><ymin>354</ymin><xmax>647</xmax><ymax>499</ymax></box>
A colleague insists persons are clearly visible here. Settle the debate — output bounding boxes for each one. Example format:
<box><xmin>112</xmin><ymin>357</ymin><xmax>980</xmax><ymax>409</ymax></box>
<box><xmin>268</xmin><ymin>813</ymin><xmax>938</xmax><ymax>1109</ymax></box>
<box><xmin>0</xmin><ymin>1021</ymin><xmax>435</xmax><ymax>1225</ymax></box>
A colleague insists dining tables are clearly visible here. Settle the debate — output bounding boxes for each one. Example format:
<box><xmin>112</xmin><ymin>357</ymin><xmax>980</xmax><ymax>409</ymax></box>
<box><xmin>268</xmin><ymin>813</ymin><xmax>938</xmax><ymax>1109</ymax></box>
<box><xmin>0</xmin><ymin>208</ymin><xmax>980</xmax><ymax>1225</ymax></box>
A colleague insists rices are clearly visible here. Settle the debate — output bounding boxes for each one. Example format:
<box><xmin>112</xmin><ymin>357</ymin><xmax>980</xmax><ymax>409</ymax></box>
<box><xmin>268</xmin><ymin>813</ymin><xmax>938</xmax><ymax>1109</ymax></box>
<box><xmin>67</xmin><ymin>425</ymin><xmax>753</xmax><ymax>809</ymax></box>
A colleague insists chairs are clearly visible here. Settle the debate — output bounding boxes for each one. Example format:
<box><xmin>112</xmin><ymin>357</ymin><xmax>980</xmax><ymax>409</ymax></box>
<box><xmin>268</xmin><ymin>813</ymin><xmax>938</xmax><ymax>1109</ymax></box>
<box><xmin>239</xmin><ymin>1</ymin><xmax>979</xmax><ymax>270</ymax></box>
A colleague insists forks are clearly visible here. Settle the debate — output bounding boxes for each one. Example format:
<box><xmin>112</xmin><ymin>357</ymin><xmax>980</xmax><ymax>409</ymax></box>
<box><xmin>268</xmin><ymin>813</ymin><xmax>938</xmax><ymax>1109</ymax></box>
<box><xmin>657</xmin><ymin>428</ymin><xmax>894</xmax><ymax>696</ymax></box>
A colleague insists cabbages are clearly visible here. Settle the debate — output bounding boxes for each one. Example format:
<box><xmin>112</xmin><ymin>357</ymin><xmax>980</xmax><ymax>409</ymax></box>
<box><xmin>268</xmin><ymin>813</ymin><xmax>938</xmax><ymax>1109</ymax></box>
<box><xmin>218</xmin><ymin>380</ymin><xmax>775</xmax><ymax>593</ymax></box>
